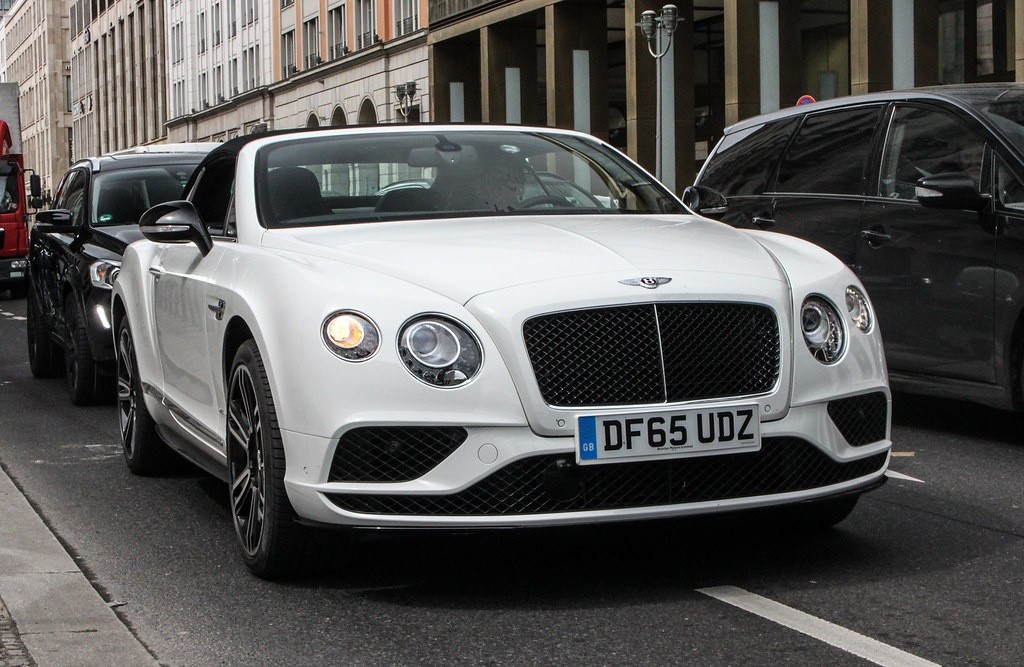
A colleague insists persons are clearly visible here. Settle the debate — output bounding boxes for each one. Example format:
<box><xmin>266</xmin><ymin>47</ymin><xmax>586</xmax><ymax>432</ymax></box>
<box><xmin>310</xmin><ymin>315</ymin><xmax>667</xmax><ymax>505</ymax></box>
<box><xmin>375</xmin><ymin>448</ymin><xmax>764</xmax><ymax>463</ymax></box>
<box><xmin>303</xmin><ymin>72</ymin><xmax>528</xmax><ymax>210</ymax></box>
<box><xmin>466</xmin><ymin>152</ymin><xmax>527</xmax><ymax>211</ymax></box>
<box><xmin>957</xmin><ymin>138</ymin><xmax>984</xmax><ymax>184</ymax></box>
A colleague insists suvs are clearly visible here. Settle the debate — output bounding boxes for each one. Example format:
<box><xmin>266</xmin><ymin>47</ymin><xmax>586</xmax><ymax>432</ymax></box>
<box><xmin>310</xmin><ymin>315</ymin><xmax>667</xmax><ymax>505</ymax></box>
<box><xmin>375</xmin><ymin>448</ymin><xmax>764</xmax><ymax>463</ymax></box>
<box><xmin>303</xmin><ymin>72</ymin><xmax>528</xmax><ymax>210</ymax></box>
<box><xmin>693</xmin><ymin>81</ymin><xmax>1024</xmax><ymax>420</ymax></box>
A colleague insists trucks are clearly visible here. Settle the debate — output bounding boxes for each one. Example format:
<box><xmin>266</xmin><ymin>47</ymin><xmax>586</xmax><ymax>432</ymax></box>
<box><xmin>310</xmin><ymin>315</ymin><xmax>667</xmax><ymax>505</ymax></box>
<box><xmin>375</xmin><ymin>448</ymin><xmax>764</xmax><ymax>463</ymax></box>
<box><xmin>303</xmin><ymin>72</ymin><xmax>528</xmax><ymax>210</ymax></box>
<box><xmin>0</xmin><ymin>121</ymin><xmax>43</xmax><ymax>300</ymax></box>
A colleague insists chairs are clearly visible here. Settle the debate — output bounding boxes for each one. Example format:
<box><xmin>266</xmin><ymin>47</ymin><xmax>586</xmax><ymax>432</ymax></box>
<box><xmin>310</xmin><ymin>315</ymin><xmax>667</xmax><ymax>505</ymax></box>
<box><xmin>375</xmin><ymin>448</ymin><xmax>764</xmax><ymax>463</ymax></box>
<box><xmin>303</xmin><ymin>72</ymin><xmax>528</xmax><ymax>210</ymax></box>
<box><xmin>267</xmin><ymin>166</ymin><xmax>336</xmax><ymax>221</ymax></box>
<box><xmin>376</xmin><ymin>188</ymin><xmax>442</xmax><ymax>212</ymax></box>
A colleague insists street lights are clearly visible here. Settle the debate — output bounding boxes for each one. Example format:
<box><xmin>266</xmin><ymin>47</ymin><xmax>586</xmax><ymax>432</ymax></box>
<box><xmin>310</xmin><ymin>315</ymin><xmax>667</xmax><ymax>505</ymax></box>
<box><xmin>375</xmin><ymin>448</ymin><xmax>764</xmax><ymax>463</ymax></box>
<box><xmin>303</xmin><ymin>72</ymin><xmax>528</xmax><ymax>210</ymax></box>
<box><xmin>634</xmin><ymin>3</ymin><xmax>686</xmax><ymax>185</ymax></box>
<box><xmin>391</xmin><ymin>80</ymin><xmax>423</xmax><ymax>123</ymax></box>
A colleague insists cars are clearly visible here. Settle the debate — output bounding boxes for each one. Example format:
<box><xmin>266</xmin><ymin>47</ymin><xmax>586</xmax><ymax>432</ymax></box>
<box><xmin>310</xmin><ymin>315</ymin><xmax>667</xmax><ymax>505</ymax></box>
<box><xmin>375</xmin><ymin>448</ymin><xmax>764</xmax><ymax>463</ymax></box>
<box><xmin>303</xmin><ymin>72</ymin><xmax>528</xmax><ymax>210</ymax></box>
<box><xmin>18</xmin><ymin>148</ymin><xmax>213</xmax><ymax>407</ymax></box>
<box><xmin>373</xmin><ymin>177</ymin><xmax>436</xmax><ymax>196</ymax></box>
<box><xmin>108</xmin><ymin>123</ymin><xmax>894</xmax><ymax>580</ymax></box>
